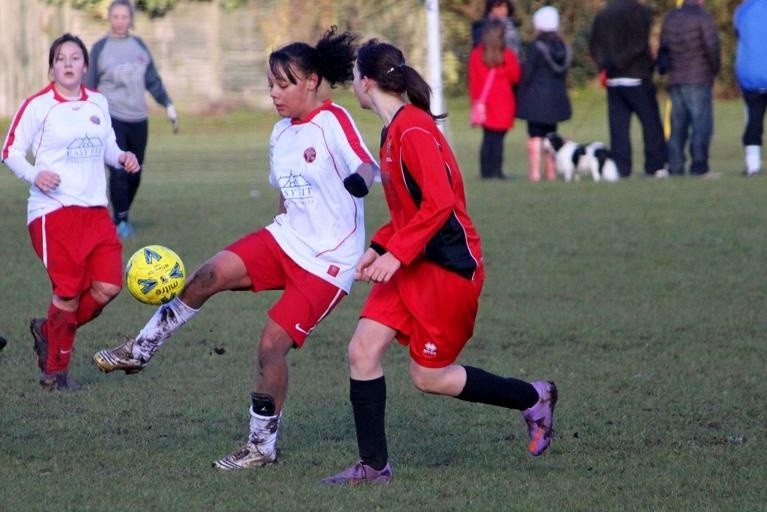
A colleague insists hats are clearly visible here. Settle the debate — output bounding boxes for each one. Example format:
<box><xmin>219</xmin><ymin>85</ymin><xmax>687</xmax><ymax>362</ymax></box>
<box><xmin>531</xmin><ymin>6</ymin><xmax>560</xmax><ymax>32</ymax></box>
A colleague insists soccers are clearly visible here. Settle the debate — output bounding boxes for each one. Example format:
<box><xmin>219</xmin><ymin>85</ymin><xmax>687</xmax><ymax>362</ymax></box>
<box><xmin>124</xmin><ymin>244</ymin><xmax>185</xmax><ymax>304</ymax></box>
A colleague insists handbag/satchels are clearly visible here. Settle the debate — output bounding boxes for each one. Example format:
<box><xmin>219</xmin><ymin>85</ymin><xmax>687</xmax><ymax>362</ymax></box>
<box><xmin>470</xmin><ymin>98</ymin><xmax>487</xmax><ymax>127</ymax></box>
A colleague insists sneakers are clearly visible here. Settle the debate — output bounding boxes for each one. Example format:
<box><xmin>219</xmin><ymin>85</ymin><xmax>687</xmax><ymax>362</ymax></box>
<box><xmin>30</xmin><ymin>317</ymin><xmax>49</xmax><ymax>372</ymax></box>
<box><xmin>38</xmin><ymin>370</ymin><xmax>70</xmax><ymax>391</ymax></box>
<box><xmin>92</xmin><ymin>334</ymin><xmax>152</xmax><ymax>375</ymax></box>
<box><xmin>211</xmin><ymin>440</ymin><xmax>278</xmax><ymax>471</ymax></box>
<box><xmin>319</xmin><ymin>460</ymin><xmax>391</xmax><ymax>487</ymax></box>
<box><xmin>116</xmin><ymin>220</ymin><xmax>132</xmax><ymax>240</ymax></box>
<box><xmin>522</xmin><ymin>380</ymin><xmax>558</xmax><ymax>457</ymax></box>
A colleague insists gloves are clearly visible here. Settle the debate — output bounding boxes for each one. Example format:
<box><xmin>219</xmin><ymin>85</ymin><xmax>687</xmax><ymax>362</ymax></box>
<box><xmin>166</xmin><ymin>104</ymin><xmax>179</xmax><ymax>134</ymax></box>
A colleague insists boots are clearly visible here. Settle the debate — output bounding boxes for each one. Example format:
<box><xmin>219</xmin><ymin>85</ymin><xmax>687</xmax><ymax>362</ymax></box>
<box><xmin>526</xmin><ymin>136</ymin><xmax>541</xmax><ymax>182</ymax></box>
<box><xmin>542</xmin><ymin>138</ymin><xmax>556</xmax><ymax>180</ymax></box>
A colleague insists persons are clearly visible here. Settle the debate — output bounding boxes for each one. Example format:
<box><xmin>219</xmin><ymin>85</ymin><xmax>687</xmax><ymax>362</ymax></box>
<box><xmin>467</xmin><ymin>16</ymin><xmax>521</xmax><ymax>181</ymax></box>
<box><xmin>658</xmin><ymin>0</ymin><xmax>722</xmax><ymax>176</ymax></box>
<box><xmin>0</xmin><ymin>34</ymin><xmax>142</xmax><ymax>385</ymax></box>
<box><xmin>510</xmin><ymin>5</ymin><xmax>571</xmax><ymax>179</ymax></box>
<box><xmin>89</xmin><ymin>22</ymin><xmax>382</xmax><ymax>470</ymax></box>
<box><xmin>83</xmin><ymin>1</ymin><xmax>178</xmax><ymax>241</ymax></box>
<box><xmin>591</xmin><ymin>0</ymin><xmax>668</xmax><ymax>177</ymax></box>
<box><xmin>315</xmin><ymin>38</ymin><xmax>557</xmax><ymax>482</ymax></box>
<box><xmin>732</xmin><ymin>0</ymin><xmax>767</xmax><ymax>177</ymax></box>
<box><xmin>467</xmin><ymin>0</ymin><xmax>528</xmax><ymax>65</ymax></box>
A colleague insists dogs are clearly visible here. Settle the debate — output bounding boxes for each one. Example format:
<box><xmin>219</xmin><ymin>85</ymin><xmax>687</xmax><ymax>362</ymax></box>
<box><xmin>541</xmin><ymin>132</ymin><xmax>620</xmax><ymax>183</ymax></box>
